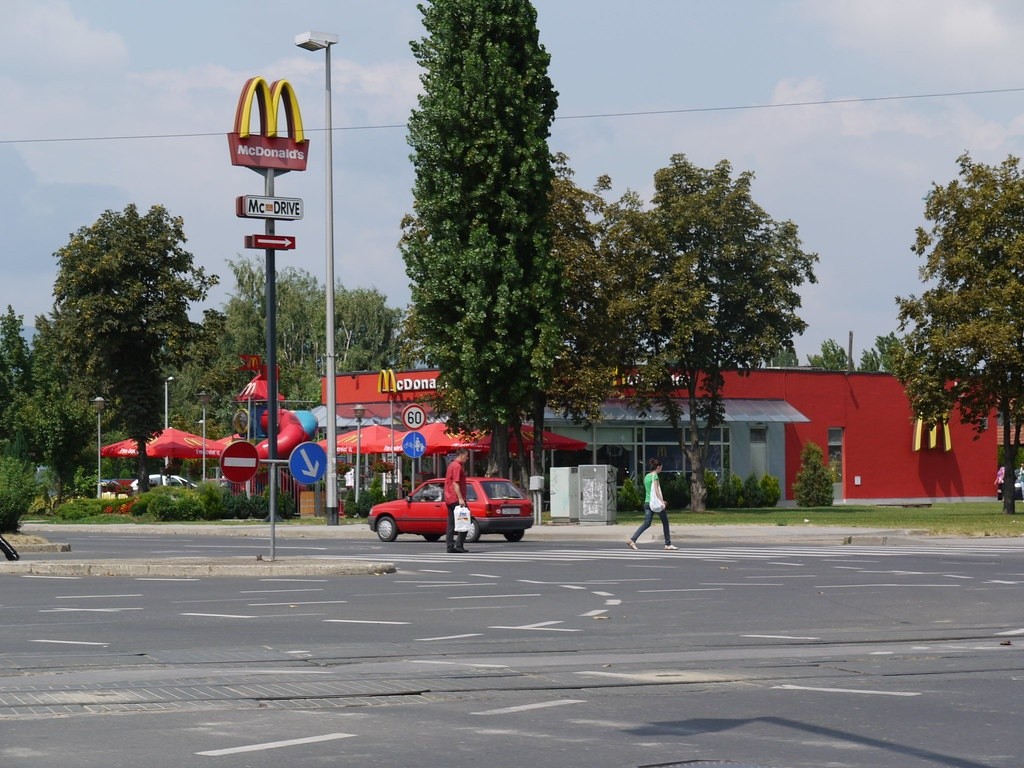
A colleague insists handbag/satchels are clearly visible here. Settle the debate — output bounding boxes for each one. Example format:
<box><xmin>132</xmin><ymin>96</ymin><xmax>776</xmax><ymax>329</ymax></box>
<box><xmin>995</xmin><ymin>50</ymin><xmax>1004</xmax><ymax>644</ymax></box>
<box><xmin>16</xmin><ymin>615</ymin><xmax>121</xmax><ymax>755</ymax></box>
<box><xmin>453</xmin><ymin>502</ymin><xmax>472</xmax><ymax>533</ymax></box>
<box><xmin>649</xmin><ymin>475</ymin><xmax>665</xmax><ymax>513</ymax></box>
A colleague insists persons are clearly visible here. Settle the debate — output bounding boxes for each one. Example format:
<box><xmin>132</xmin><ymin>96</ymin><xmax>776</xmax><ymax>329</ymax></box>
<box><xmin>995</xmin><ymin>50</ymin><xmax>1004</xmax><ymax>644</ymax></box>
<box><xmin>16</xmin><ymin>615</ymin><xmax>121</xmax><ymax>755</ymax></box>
<box><xmin>1017</xmin><ymin>462</ymin><xmax>1024</xmax><ymax>502</ymax></box>
<box><xmin>627</xmin><ymin>459</ymin><xmax>678</xmax><ymax>549</ymax></box>
<box><xmin>994</xmin><ymin>464</ymin><xmax>1006</xmax><ymax>501</ymax></box>
<box><xmin>444</xmin><ymin>448</ymin><xmax>472</xmax><ymax>553</ymax></box>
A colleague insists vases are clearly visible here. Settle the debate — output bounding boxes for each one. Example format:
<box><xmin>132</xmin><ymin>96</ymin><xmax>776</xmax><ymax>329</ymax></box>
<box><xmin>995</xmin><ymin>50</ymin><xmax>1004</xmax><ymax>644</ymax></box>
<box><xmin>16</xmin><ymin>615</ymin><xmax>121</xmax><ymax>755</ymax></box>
<box><xmin>414</xmin><ymin>473</ymin><xmax>435</xmax><ymax>484</ymax></box>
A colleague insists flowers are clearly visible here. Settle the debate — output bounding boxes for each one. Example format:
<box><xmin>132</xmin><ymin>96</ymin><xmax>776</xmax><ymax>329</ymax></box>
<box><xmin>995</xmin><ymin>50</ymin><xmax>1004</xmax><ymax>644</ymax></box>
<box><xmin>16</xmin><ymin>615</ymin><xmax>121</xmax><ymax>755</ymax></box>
<box><xmin>417</xmin><ymin>470</ymin><xmax>433</xmax><ymax>474</ymax></box>
<box><xmin>336</xmin><ymin>460</ymin><xmax>355</xmax><ymax>476</ymax></box>
<box><xmin>369</xmin><ymin>459</ymin><xmax>396</xmax><ymax>472</ymax></box>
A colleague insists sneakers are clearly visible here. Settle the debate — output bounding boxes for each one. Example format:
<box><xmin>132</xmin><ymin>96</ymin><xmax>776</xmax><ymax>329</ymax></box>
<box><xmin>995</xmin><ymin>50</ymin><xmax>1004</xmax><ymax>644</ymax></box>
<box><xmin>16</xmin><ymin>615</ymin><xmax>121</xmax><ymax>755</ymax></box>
<box><xmin>664</xmin><ymin>544</ymin><xmax>678</xmax><ymax>550</ymax></box>
<box><xmin>626</xmin><ymin>538</ymin><xmax>639</xmax><ymax>550</ymax></box>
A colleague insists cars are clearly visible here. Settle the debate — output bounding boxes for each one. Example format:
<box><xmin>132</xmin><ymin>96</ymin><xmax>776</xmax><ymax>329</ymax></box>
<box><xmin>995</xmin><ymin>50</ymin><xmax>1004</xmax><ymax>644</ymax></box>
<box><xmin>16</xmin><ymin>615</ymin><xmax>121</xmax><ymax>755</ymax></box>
<box><xmin>130</xmin><ymin>473</ymin><xmax>198</xmax><ymax>492</ymax></box>
<box><xmin>999</xmin><ymin>468</ymin><xmax>1022</xmax><ymax>499</ymax></box>
<box><xmin>368</xmin><ymin>478</ymin><xmax>534</xmax><ymax>542</ymax></box>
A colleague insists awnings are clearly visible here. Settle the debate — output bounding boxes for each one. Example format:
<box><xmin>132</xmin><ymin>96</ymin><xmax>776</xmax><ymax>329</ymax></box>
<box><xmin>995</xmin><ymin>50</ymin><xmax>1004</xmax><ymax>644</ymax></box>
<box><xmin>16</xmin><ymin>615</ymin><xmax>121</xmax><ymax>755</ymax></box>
<box><xmin>523</xmin><ymin>398</ymin><xmax>812</xmax><ymax>426</ymax></box>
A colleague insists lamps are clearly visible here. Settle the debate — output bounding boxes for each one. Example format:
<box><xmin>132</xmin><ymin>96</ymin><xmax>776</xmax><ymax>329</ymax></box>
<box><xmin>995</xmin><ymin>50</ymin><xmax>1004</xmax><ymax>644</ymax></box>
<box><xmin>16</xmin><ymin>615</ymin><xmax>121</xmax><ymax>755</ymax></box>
<box><xmin>844</xmin><ymin>369</ymin><xmax>852</xmax><ymax>377</ymax></box>
<box><xmin>351</xmin><ymin>372</ymin><xmax>357</xmax><ymax>379</ymax></box>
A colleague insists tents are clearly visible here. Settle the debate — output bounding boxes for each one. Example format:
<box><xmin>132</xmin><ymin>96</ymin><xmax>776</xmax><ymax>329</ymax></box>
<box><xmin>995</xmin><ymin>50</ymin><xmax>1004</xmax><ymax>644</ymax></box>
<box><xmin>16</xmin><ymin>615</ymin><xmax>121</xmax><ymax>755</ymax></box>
<box><xmin>437</xmin><ymin>423</ymin><xmax>587</xmax><ymax>481</ymax></box>
<box><xmin>367</xmin><ymin>421</ymin><xmax>493</xmax><ymax>495</ymax></box>
<box><xmin>100</xmin><ymin>429</ymin><xmax>244</xmax><ymax>475</ymax></box>
<box><xmin>313</xmin><ymin>425</ymin><xmax>402</xmax><ymax>503</ymax></box>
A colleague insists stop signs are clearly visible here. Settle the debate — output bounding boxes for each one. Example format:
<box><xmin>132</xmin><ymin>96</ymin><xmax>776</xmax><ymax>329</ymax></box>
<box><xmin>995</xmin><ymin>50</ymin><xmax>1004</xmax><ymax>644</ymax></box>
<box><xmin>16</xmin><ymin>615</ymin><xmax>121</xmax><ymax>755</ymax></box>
<box><xmin>220</xmin><ymin>441</ymin><xmax>259</xmax><ymax>483</ymax></box>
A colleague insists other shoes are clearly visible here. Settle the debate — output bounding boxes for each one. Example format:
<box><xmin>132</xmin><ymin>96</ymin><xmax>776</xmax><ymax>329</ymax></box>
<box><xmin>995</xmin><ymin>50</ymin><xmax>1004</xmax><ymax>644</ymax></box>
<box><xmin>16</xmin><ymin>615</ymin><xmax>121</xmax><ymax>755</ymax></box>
<box><xmin>447</xmin><ymin>547</ymin><xmax>455</xmax><ymax>552</ymax></box>
<box><xmin>452</xmin><ymin>546</ymin><xmax>469</xmax><ymax>553</ymax></box>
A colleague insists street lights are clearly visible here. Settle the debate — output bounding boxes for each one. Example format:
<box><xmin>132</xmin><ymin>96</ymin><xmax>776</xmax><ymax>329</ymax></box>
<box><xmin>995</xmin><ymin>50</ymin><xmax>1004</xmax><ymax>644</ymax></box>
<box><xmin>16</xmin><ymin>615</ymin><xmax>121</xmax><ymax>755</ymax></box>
<box><xmin>164</xmin><ymin>376</ymin><xmax>174</xmax><ymax>487</ymax></box>
<box><xmin>352</xmin><ymin>405</ymin><xmax>365</xmax><ymax>518</ymax></box>
<box><xmin>195</xmin><ymin>391</ymin><xmax>205</xmax><ymax>483</ymax></box>
<box><xmin>90</xmin><ymin>397</ymin><xmax>106</xmax><ymax>500</ymax></box>
<box><xmin>296</xmin><ymin>32</ymin><xmax>339</xmax><ymax>527</ymax></box>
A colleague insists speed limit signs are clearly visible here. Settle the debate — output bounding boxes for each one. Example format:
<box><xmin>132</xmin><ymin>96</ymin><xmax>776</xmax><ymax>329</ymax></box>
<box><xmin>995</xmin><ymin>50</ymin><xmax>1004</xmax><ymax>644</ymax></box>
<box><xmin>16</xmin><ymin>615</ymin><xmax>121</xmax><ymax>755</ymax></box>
<box><xmin>402</xmin><ymin>404</ymin><xmax>427</xmax><ymax>430</ymax></box>
